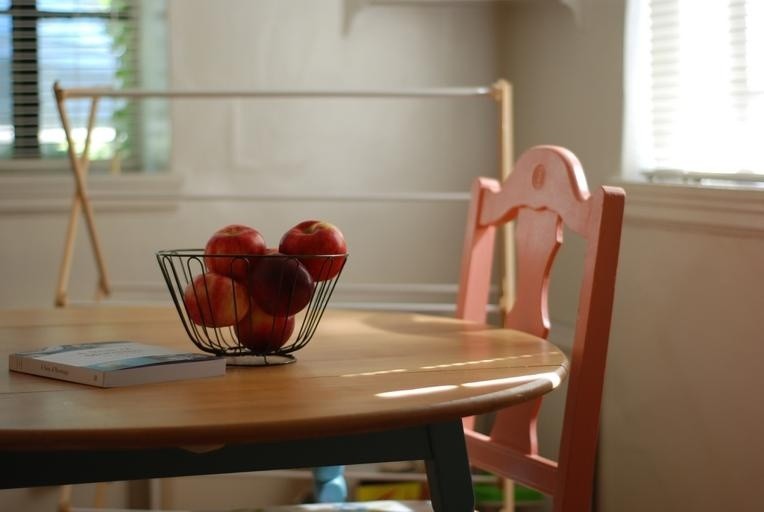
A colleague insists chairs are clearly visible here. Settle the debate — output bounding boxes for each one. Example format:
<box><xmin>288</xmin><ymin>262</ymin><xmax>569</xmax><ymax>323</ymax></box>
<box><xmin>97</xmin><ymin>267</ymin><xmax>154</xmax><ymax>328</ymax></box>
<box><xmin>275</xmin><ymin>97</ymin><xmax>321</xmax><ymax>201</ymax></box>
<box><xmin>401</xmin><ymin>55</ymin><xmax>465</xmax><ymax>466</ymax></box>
<box><xmin>454</xmin><ymin>141</ymin><xmax>630</xmax><ymax>511</ymax></box>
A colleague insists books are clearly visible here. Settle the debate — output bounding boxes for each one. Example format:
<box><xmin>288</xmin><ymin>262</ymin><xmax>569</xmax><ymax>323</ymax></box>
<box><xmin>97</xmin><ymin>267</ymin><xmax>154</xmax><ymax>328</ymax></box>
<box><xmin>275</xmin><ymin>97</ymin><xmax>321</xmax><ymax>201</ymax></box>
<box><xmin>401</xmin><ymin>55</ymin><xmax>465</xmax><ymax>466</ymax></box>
<box><xmin>9</xmin><ymin>341</ymin><xmax>226</xmax><ymax>388</ymax></box>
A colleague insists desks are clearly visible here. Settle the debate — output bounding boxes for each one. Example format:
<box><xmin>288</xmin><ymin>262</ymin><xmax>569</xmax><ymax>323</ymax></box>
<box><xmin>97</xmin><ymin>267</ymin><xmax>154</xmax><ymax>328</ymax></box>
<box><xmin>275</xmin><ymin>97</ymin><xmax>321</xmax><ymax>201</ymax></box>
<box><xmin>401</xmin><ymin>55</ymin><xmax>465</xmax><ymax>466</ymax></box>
<box><xmin>0</xmin><ymin>302</ymin><xmax>573</xmax><ymax>510</ymax></box>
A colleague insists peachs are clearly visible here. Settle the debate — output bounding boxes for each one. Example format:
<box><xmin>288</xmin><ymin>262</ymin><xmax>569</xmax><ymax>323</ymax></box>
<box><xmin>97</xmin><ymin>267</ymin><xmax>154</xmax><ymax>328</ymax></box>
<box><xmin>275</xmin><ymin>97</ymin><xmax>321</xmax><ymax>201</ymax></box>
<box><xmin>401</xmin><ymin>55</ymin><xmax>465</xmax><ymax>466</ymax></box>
<box><xmin>184</xmin><ymin>220</ymin><xmax>347</xmax><ymax>352</ymax></box>
